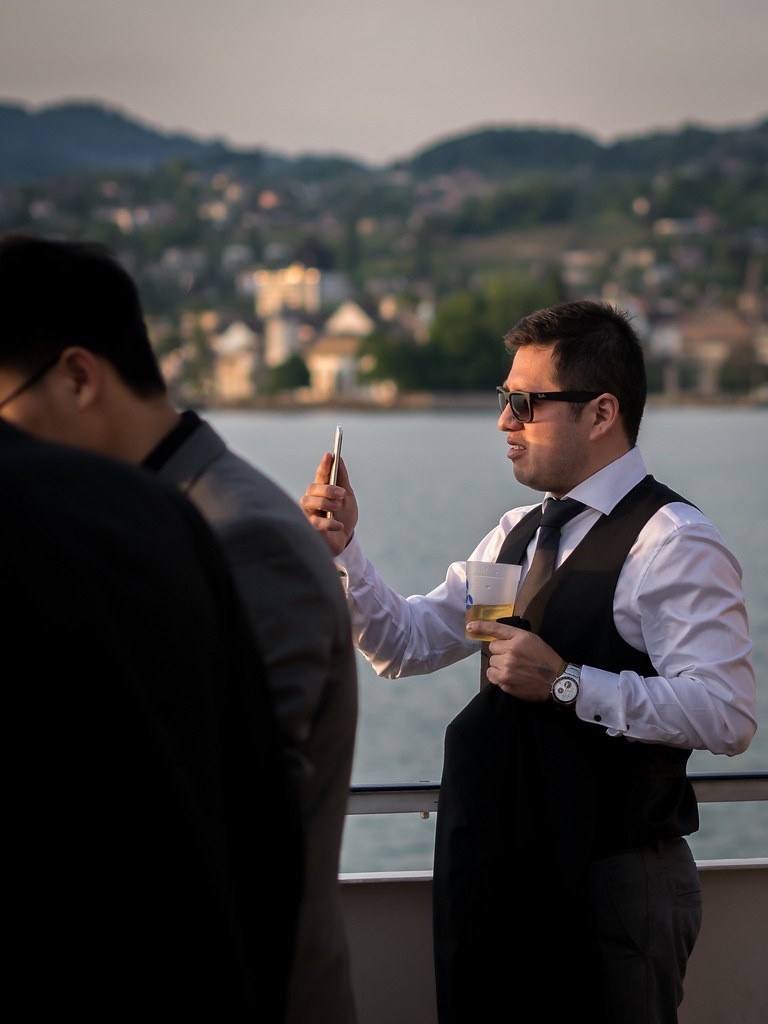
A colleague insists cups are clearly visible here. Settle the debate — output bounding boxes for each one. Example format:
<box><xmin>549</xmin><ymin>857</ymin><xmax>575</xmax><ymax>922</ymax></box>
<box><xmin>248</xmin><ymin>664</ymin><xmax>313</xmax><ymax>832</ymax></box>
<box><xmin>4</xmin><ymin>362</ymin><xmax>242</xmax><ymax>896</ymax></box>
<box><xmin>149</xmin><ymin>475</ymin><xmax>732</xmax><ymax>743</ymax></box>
<box><xmin>465</xmin><ymin>560</ymin><xmax>523</xmax><ymax>641</ymax></box>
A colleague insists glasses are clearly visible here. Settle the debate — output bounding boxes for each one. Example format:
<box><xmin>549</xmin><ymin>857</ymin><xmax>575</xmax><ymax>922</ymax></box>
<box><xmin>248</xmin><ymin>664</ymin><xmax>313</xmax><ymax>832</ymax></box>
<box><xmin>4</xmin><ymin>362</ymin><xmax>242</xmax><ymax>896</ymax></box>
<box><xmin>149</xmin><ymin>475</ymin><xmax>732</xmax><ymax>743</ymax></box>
<box><xmin>495</xmin><ymin>385</ymin><xmax>599</xmax><ymax>423</ymax></box>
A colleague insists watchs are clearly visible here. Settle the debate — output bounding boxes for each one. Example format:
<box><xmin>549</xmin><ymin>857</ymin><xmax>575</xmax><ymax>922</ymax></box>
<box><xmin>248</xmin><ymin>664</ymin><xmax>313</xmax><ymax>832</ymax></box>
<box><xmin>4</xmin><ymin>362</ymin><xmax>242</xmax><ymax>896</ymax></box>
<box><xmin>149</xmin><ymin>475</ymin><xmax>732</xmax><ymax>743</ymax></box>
<box><xmin>549</xmin><ymin>663</ymin><xmax>581</xmax><ymax>707</ymax></box>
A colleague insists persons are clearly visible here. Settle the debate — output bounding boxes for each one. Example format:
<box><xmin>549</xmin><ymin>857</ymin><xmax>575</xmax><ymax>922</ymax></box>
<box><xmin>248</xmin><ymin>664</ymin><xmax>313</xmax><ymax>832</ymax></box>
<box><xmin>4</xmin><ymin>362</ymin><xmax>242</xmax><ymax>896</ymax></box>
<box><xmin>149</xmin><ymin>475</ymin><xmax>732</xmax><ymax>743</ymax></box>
<box><xmin>301</xmin><ymin>303</ymin><xmax>757</xmax><ymax>1024</ymax></box>
<box><xmin>1</xmin><ymin>234</ymin><xmax>365</xmax><ymax>1024</ymax></box>
<box><xmin>0</xmin><ymin>418</ymin><xmax>304</xmax><ymax>1024</ymax></box>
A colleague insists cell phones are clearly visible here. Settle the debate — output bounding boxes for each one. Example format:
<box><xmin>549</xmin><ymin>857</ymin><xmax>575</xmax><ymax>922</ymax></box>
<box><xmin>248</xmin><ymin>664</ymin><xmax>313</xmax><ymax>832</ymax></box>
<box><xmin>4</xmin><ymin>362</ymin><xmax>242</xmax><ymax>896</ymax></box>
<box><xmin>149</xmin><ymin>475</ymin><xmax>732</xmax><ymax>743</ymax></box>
<box><xmin>327</xmin><ymin>423</ymin><xmax>343</xmax><ymax>519</ymax></box>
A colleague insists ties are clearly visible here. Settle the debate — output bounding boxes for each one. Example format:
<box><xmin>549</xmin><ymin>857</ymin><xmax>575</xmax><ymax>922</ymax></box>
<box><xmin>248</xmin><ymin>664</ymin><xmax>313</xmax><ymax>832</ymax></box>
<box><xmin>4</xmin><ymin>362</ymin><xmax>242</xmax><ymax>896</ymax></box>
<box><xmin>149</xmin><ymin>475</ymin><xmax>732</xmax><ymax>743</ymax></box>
<box><xmin>512</xmin><ymin>496</ymin><xmax>590</xmax><ymax>619</ymax></box>
